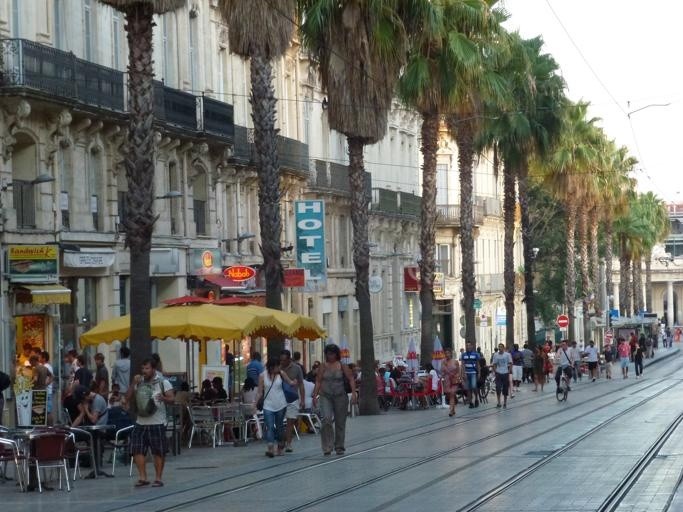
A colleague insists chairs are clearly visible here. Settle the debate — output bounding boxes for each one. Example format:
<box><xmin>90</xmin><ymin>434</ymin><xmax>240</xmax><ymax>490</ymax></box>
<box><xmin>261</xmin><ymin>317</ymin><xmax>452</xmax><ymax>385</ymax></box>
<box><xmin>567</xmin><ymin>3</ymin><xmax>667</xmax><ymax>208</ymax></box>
<box><xmin>348</xmin><ymin>358</ymin><xmax>446</xmax><ymax>416</ymax></box>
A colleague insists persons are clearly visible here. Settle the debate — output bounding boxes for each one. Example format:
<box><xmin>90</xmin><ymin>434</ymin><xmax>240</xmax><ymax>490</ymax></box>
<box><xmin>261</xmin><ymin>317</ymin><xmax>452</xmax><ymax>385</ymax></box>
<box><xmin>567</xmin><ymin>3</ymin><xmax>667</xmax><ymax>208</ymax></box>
<box><xmin>241</xmin><ymin>377</ymin><xmax>265</xmax><ymax>432</ymax></box>
<box><xmin>342</xmin><ymin>360</ymin><xmax>362</xmax><ymax>393</ymax></box>
<box><xmin>200</xmin><ymin>379</ymin><xmax>215</xmax><ymax>406</ymax></box>
<box><xmin>226</xmin><ymin>344</ymin><xmax>234</xmax><ymax>401</ymax></box>
<box><xmin>246</xmin><ymin>352</ymin><xmax>264</xmax><ymax>386</ymax></box>
<box><xmin>277</xmin><ymin>350</ymin><xmax>305</xmax><ymax>452</ymax></box>
<box><xmin>661</xmin><ymin>327</ymin><xmax>683</xmax><ymax>348</ymax></box>
<box><xmin>256</xmin><ymin>355</ymin><xmax>297</xmax><ymax>457</ymax></box>
<box><xmin>307</xmin><ymin>361</ymin><xmax>320</xmax><ymax>384</ymax></box>
<box><xmin>0</xmin><ymin>371</ymin><xmax>10</xmax><ymax>425</ymax></box>
<box><xmin>292</xmin><ymin>352</ymin><xmax>305</xmax><ymax>379</ymax></box>
<box><xmin>14</xmin><ymin>341</ymin><xmax>175</xmax><ymax>487</ymax></box>
<box><xmin>175</xmin><ymin>382</ymin><xmax>193</xmax><ymax>439</ymax></box>
<box><xmin>554</xmin><ymin>340</ymin><xmax>613</xmax><ymax>393</ymax></box>
<box><xmin>441</xmin><ymin>341</ymin><xmax>552</xmax><ymax>416</ymax></box>
<box><xmin>374</xmin><ymin>359</ymin><xmax>439</xmax><ymax>410</ymax></box>
<box><xmin>312</xmin><ymin>343</ymin><xmax>356</xmax><ymax>455</ymax></box>
<box><xmin>617</xmin><ymin>332</ymin><xmax>657</xmax><ymax>379</ymax></box>
<box><xmin>212</xmin><ymin>377</ymin><xmax>227</xmax><ymax>403</ymax></box>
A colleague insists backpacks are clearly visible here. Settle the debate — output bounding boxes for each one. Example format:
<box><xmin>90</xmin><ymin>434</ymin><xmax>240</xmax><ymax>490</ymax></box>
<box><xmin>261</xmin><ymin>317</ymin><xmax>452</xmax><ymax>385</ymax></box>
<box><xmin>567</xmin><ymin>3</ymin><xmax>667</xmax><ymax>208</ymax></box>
<box><xmin>134</xmin><ymin>377</ymin><xmax>164</xmax><ymax>416</ymax></box>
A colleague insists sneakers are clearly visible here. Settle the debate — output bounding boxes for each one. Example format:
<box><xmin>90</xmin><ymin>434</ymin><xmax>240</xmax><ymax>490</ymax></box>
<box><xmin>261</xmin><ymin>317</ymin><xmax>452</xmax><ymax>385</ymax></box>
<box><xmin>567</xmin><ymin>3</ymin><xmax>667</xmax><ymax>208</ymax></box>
<box><xmin>266</xmin><ymin>446</ymin><xmax>344</xmax><ymax>457</ymax></box>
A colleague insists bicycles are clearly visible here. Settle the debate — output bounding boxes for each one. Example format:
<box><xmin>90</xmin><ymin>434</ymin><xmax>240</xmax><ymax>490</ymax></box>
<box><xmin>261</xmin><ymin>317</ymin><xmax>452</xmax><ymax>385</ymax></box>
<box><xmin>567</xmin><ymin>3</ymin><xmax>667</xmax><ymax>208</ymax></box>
<box><xmin>477</xmin><ymin>374</ymin><xmax>503</xmax><ymax>399</ymax></box>
<box><xmin>553</xmin><ymin>366</ymin><xmax>570</xmax><ymax>403</ymax></box>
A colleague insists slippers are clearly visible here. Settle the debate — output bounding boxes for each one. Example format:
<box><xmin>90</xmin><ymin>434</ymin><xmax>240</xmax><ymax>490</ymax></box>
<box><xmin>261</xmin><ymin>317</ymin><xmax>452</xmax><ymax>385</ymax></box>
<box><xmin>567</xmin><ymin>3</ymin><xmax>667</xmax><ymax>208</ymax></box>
<box><xmin>136</xmin><ymin>480</ymin><xmax>149</xmax><ymax>486</ymax></box>
<box><xmin>151</xmin><ymin>481</ymin><xmax>163</xmax><ymax>487</ymax></box>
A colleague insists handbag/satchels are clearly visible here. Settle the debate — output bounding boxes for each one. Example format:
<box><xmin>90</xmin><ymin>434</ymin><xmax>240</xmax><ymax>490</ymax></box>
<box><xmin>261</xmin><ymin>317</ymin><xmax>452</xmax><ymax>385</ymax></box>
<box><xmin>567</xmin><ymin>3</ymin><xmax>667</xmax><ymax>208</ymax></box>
<box><xmin>283</xmin><ymin>382</ymin><xmax>299</xmax><ymax>402</ymax></box>
<box><xmin>257</xmin><ymin>399</ymin><xmax>263</xmax><ymax>410</ymax></box>
<box><xmin>343</xmin><ymin>375</ymin><xmax>352</xmax><ymax>393</ymax></box>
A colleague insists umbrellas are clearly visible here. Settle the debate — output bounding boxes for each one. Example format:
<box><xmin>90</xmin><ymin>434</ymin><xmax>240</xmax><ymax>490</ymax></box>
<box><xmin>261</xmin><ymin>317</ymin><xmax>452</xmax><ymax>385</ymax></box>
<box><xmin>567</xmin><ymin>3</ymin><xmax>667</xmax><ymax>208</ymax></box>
<box><xmin>80</xmin><ymin>291</ymin><xmax>328</xmax><ymax>387</ymax></box>
